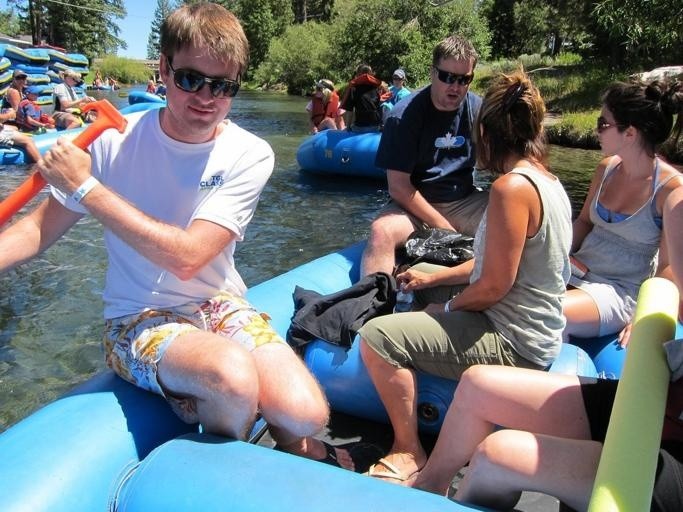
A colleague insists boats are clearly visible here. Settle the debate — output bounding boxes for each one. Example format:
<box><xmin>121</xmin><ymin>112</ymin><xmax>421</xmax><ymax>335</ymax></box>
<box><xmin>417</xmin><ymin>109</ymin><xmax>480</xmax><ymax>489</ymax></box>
<box><xmin>0</xmin><ymin>34</ymin><xmax>171</xmax><ymax>167</ymax></box>
<box><xmin>295</xmin><ymin>122</ymin><xmax>475</xmax><ymax>191</ymax></box>
<box><xmin>1</xmin><ymin>234</ymin><xmax>682</xmax><ymax>507</ymax></box>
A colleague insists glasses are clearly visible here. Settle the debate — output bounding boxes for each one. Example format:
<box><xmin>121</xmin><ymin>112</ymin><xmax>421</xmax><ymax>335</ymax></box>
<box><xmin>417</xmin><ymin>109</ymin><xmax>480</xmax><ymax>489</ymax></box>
<box><xmin>433</xmin><ymin>65</ymin><xmax>474</xmax><ymax>86</ymax></box>
<box><xmin>597</xmin><ymin>117</ymin><xmax>625</xmax><ymax>133</ymax></box>
<box><xmin>166</xmin><ymin>55</ymin><xmax>241</xmax><ymax>100</ymax></box>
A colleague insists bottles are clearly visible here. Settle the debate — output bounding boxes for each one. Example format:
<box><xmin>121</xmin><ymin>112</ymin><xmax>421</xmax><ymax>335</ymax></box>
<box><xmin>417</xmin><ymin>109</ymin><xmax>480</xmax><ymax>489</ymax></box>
<box><xmin>392</xmin><ymin>281</ymin><xmax>417</xmax><ymax>314</ymax></box>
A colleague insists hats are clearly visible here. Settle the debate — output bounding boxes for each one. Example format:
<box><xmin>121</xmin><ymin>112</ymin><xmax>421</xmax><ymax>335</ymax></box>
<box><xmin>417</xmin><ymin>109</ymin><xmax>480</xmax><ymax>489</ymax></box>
<box><xmin>392</xmin><ymin>69</ymin><xmax>405</xmax><ymax>79</ymax></box>
<box><xmin>12</xmin><ymin>69</ymin><xmax>27</xmax><ymax>78</ymax></box>
<box><xmin>23</xmin><ymin>85</ymin><xmax>44</xmax><ymax>94</ymax></box>
<box><xmin>315</xmin><ymin>81</ymin><xmax>333</xmax><ymax>91</ymax></box>
<box><xmin>64</xmin><ymin>73</ymin><xmax>84</xmax><ymax>82</ymax></box>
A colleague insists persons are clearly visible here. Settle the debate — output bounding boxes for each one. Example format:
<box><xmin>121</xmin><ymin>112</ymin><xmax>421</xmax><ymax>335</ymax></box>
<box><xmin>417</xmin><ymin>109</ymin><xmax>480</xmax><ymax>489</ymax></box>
<box><xmin>0</xmin><ymin>0</ymin><xmax>355</xmax><ymax>472</ymax></box>
<box><xmin>563</xmin><ymin>79</ymin><xmax>683</xmax><ymax>350</ymax></box>
<box><xmin>306</xmin><ymin>64</ymin><xmax>411</xmax><ymax>134</ymax></box>
<box><xmin>398</xmin><ymin>339</ymin><xmax>683</xmax><ymax>512</ymax></box>
<box><xmin>357</xmin><ymin>59</ymin><xmax>573</xmax><ymax>484</ymax></box>
<box><xmin>0</xmin><ymin>69</ymin><xmax>167</xmax><ymax>164</ymax></box>
<box><xmin>360</xmin><ymin>36</ymin><xmax>490</xmax><ymax>282</ymax></box>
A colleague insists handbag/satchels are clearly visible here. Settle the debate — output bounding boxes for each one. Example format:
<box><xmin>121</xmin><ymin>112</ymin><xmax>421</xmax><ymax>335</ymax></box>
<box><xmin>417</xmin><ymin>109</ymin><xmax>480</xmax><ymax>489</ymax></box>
<box><xmin>392</xmin><ymin>227</ymin><xmax>474</xmax><ymax>278</ymax></box>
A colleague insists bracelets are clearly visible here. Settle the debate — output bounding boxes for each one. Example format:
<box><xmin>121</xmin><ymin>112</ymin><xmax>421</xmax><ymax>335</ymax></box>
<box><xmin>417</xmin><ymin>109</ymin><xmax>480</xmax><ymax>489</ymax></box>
<box><xmin>72</xmin><ymin>176</ymin><xmax>100</xmax><ymax>204</ymax></box>
<box><xmin>445</xmin><ymin>299</ymin><xmax>452</xmax><ymax>313</ymax></box>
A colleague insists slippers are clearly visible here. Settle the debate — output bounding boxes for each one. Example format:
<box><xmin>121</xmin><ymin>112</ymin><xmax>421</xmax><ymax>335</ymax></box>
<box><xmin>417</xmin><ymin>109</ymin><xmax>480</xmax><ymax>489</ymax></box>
<box><xmin>360</xmin><ymin>457</ymin><xmax>468</xmax><ymax>499</ymax></box>
<box><xmin>273</xmin><ymin>440</ymin><xmax>342</xmax><ymax>468</ymax></box>
